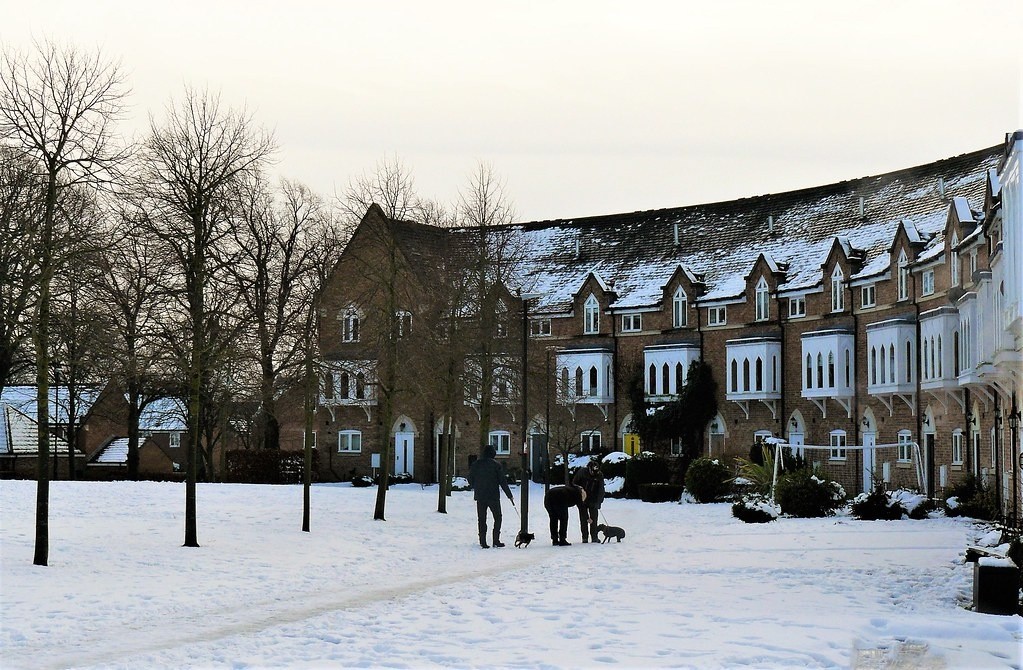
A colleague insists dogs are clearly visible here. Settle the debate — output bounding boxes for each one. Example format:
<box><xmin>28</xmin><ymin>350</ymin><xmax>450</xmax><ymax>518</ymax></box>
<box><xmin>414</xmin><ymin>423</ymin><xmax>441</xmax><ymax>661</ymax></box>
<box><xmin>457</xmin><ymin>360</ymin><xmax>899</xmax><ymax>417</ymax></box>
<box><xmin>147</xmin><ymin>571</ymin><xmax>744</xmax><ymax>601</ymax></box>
<box><xmin>514</xmin><ymin>530</ymin><xmax>535</xmax><ymax>549</ymax></box>
<box><xmin>596</xmin><ymin>523</ymin><xmax>625</xmax><ymax>544</ymax></box>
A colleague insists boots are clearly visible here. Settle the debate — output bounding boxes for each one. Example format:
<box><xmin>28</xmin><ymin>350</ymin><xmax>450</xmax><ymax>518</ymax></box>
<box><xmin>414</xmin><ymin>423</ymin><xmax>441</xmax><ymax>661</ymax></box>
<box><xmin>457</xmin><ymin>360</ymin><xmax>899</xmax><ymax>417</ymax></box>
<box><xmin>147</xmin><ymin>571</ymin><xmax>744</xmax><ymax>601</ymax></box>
<box><xmin>478</xmin><ymin>532</ymin><xmax>490</xmax><ymax>548</ymax></box>
<box><xmin>492</xmin><ymin>529</ymin><xmax>505</xmax><ymax>547</ymax></box>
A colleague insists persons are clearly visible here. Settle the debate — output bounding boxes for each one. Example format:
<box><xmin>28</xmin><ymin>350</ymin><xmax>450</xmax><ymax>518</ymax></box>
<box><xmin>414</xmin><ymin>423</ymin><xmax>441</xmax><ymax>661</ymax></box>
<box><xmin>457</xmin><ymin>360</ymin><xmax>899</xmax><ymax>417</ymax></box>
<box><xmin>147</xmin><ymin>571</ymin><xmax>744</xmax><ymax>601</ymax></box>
<box><xmin>466</xmin><ymin>444</ymin><xmax>514</xmax><ymax>549</ymax></box>
<box><xmin>573</xmin><ymin>461</ymin><xmax>605</xmax><ymax>543</ymax></box>
<box><xmin>544</xmin><ymin>482</ymin><xmax>593</xmax><ymax>547</ymax></box>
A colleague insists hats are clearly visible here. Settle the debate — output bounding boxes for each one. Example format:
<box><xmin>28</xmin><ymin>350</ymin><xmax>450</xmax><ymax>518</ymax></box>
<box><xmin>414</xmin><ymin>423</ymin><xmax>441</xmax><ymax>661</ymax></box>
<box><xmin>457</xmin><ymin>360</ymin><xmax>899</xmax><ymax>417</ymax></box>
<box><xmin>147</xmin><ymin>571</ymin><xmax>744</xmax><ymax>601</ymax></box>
<box><xmin>579</xmin><ymin>486</ymin><xmax>586</xmax><ymax>502</ymax></box>
<box><xmin>589</xmin><ymin>461</ymin><xmax>600</xmax><ymax>473</ymax></box>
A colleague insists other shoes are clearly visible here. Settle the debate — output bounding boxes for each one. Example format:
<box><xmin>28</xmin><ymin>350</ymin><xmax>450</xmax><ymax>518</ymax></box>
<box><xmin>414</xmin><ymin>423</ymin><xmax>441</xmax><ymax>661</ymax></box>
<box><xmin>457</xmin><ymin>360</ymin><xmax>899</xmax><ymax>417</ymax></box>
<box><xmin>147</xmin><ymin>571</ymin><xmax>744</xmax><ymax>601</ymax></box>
<box><xmin>552</xmin><ymin>541</ymin><xmax>560</xmax><ymax>545</ymax></box>
<box><xmin>559</xmin><ymin>539</ymin><xmax>570</xmax><ymax>546</ymax></box>
<box><xmin>581</xmin><ymin>538</ymin><xmax>588</xmax><ymax>543</ymax></box>
<box><xmin>592</xmin><ymin>538</ymin><xmax>601</xmax><ymax>543</ymax></box>
